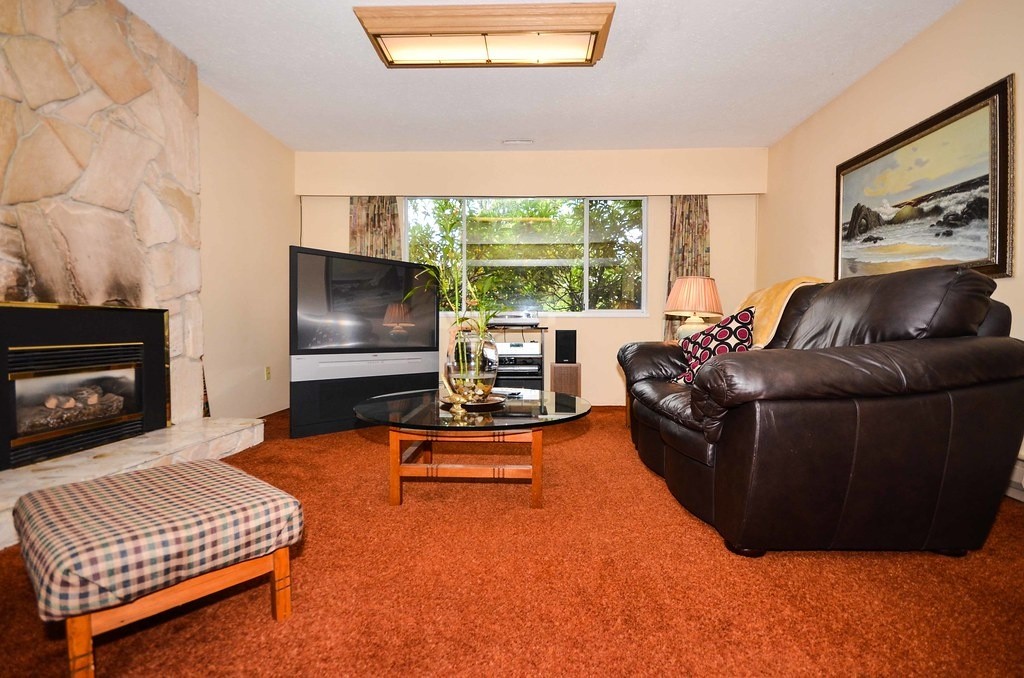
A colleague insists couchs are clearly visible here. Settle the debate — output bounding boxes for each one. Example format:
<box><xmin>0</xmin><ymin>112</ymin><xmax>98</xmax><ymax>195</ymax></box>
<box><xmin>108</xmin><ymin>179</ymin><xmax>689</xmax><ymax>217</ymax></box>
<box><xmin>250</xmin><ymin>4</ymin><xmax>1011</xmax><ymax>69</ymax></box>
<box><xmin>615</xmin><ymin>267</ymin><xmax>1024</xmax><ymax>558</ymax></box>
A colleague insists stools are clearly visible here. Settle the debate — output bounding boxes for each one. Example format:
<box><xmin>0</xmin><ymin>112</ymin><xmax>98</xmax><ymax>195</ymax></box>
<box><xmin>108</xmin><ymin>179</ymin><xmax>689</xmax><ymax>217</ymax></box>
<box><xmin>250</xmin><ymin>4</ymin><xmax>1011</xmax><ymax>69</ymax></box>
<box><xmin>13</xmin><ymin>458</ymin><xmax>303</xmax><ymax>678</ymax></box>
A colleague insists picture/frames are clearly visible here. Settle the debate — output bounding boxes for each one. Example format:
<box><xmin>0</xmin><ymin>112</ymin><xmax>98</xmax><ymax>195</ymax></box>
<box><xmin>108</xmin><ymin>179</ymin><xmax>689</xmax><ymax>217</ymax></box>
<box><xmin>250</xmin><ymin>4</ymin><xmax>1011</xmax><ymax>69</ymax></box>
<box><xmin>833</xmin><ymin>72</ymin><xmax>1017</xmax><ymax>286</ymax></box>
<box><xmin>324</xmin><ymin>256</ymin><xmax>412</xmax><ymax>319</ymax></box>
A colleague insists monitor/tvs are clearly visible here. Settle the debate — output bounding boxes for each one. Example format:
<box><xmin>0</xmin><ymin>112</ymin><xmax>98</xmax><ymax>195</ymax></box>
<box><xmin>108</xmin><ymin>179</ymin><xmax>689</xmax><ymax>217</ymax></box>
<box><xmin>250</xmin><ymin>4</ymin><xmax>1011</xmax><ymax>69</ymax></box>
<box><xmin>286</xmin><ymin>245</ymin><xmax>439</xmax><ymax>355</ymax></box>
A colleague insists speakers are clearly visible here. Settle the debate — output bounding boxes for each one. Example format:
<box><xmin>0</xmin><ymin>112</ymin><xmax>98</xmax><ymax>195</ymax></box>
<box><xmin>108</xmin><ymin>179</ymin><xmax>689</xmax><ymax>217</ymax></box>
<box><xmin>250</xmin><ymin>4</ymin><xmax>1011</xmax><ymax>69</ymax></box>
<box><xmin>555</xmin><ymin>329</ymin><xmax>577</xmax><ymax>363</ymax></box>
<box><xmin>555</xmin><ymin>392</ymin><xmax>576</xmax><ymax>414</ymax></box>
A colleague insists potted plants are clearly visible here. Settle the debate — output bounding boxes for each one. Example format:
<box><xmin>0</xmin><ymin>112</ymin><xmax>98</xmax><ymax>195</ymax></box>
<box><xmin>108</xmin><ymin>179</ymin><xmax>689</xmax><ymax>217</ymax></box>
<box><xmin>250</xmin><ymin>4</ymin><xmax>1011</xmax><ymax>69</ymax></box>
<box><xmin>401</xmin><ymin>197</ymin><xmax>519</xmax><ymax>401</ymax></box>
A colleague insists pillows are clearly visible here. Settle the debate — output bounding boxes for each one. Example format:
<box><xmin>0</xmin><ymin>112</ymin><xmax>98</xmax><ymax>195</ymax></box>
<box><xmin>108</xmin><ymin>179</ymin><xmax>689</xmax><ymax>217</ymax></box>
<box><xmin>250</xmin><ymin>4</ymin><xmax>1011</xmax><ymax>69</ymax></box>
<box><xmin>667</xmin><ymin>305</ymin><xmax>757</xmax><ymax>385</ymax></box>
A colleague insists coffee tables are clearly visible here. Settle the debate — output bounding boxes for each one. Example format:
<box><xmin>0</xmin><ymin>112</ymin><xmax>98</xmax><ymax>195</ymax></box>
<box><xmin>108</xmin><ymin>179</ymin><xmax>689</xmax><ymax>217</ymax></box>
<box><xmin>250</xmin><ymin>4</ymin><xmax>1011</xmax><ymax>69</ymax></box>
<box><xmin>352</xmin><ymin>384</ymin><xmax>592</xmax><ymax>511</ymax></box>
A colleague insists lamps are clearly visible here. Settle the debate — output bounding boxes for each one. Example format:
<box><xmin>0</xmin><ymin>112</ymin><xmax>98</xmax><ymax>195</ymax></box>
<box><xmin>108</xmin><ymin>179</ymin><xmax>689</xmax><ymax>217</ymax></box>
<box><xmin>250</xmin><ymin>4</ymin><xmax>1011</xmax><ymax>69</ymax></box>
<box><xmin>352</xmin><ymin>2</ymin><xmax>618</xmax><ymax>70</ymax></box>
<box><xmin>662</xmin><ymin>274</ymin><xmax>724</xmax><ymax>341</ymax></box>
<box><xmin>381</xmin><ymin>302</ymin><xmax>416</xmax><ymax>347</ymax></box>
